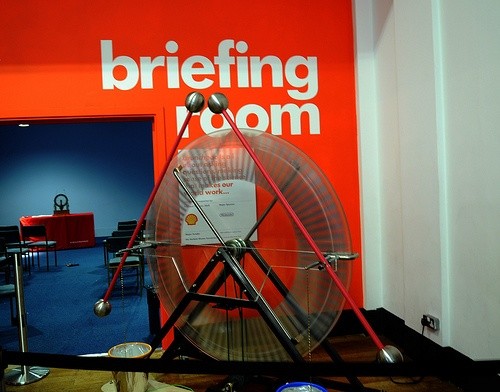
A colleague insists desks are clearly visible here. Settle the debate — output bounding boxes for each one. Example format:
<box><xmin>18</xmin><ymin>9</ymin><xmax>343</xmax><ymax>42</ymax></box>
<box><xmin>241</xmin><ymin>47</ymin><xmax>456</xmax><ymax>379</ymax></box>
<box><xmin>19</xmin><ymin>212</ymin><xmax>95</xmax><ymax>251</ymax></box>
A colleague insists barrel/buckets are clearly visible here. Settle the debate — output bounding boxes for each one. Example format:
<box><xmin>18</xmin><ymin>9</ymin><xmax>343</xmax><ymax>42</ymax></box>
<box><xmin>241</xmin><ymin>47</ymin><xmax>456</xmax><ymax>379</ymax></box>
<box><xmin>275</xmin><ymin>381</ymin><xmax>327</xmax><ymax>392</ymax></box>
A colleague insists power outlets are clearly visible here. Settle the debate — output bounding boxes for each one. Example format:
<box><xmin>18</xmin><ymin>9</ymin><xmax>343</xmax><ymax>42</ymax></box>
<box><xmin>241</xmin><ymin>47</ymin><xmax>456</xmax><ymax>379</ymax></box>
<box><xmin>422</xmin><ymin>315</ymin><xmax>439</xmax><ymax>330</ymax></box>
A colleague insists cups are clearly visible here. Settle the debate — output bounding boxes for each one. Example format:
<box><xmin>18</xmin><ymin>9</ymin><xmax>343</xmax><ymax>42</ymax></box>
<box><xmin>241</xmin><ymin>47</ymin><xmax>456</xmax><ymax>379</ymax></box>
<box><xmin>110</xmin><ymin>342</ymin><xmax>153</xmax><ymax>391</ymax></box>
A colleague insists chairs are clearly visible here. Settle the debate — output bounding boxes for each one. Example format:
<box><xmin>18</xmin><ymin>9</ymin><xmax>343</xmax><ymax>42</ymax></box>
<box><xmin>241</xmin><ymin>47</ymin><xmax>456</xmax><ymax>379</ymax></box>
<box><xmin>103</xmin><ymin>220</ymin><xmax>146</xmax><ymax>300</ymax></box>
<box><xmin>0</xmin><ymin>225</ymin><xmax>57</xmax><ymax>328</ymax></box>
